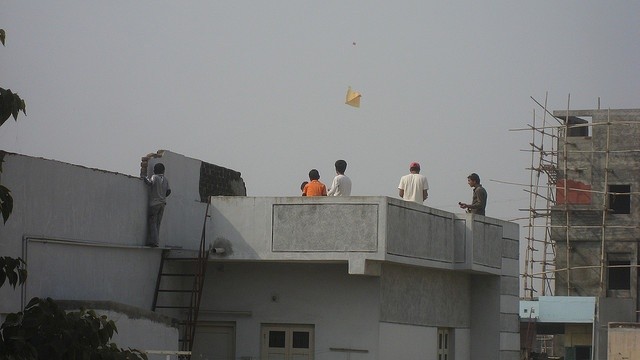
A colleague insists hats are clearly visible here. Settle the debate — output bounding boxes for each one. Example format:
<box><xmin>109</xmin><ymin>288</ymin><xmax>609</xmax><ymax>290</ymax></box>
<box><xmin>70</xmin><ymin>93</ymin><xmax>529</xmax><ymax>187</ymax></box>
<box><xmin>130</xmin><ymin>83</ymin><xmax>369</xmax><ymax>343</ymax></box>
<box><xmin>410</xmin><ymin>162</ymin><xmax>420</xmax><ymax>168</ymax></box>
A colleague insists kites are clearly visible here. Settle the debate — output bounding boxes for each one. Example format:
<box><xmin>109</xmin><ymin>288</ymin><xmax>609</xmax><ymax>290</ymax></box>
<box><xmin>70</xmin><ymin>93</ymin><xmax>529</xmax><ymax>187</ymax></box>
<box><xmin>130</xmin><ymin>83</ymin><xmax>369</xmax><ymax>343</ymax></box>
<box><xmin>345</xmin><ymin>85</ymin><xmax>362</xmax><ymax>108</ymax></box>
<box><xmin>352</xmin><ymin>41</ymin><xmax>356</xmax><ymax>46</ymax></box>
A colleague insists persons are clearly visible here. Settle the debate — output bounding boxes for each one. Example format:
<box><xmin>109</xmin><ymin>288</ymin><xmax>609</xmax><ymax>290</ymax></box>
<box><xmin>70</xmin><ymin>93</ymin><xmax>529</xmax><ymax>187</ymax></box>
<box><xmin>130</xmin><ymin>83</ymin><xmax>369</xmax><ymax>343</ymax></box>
<box><xmin>143</xmin><ymin>163</ymin><xmax>171</xmax><ymax>247</ymax></box>
<box><xmin>327</xmin><ymin>160</ymin><xmax>352</xmax><ymax>196</ymax></box>
<box><xmin>301</xmin><ymin>182</ymin><xmax>308</xmax><ymax>190</ymax></box>
<box><xmin>459</xmin><ymin>173</ymin><xmax>487</xmax><ymax>216</ymax></box>
<box><xmin>398</xmin><ymin>162</ymin><xmax>429</xmax><ymax>204</ymax></box>
<box><xmin>302</xmin><ymin>169</ymin><xmax>327</xmax><ymax>196</ymax></box>
